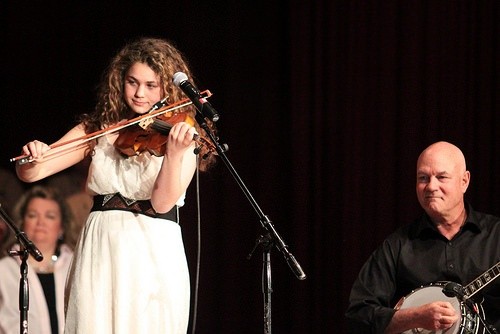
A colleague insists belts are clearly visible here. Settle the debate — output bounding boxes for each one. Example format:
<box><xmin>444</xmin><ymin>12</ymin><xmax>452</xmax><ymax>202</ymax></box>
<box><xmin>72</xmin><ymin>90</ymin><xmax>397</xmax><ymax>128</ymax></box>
<box><xmin>90</xmin><ymin>191</ymin><xmax>178</xmax><ymax>224</ymax></box>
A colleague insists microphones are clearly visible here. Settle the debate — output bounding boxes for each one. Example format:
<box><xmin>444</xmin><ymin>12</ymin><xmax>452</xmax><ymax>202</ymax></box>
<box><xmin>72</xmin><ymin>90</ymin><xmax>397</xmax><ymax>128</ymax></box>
<box><xmin>443</xmin><ymin>282</ymin><xmax>478</xmax><ymax>313</ymax></box>
<box><xmin>172</xmin><ymin>71</ymin><xmax>220</xmax><ymax>122</ymax></box>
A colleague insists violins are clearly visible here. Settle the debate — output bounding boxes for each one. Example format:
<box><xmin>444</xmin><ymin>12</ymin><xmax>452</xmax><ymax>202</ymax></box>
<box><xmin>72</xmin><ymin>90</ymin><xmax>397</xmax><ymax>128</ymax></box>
<box><xmin>113</xmin><ymin>111</ymin><xmax>227</xmax><ymax>160</ymax></box>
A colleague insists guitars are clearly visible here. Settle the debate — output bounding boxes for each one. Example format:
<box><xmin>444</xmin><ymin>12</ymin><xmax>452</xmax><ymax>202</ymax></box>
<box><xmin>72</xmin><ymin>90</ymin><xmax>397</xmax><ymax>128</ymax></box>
<box><xmin>393</xmin><ymin>259</ymin><xmax>500</xmax><ymax>333</ymax></box>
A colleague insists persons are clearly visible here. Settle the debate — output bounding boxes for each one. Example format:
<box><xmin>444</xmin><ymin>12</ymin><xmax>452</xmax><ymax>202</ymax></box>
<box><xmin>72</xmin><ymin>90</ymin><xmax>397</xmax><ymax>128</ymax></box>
<box><xmin>15</xmin><ymin>38</ymin><xmax>200</xmax><ymax>334</ymax></box>
<box><xmin>0</xmin><ymin>184</ymin><xmax>76</xmax><ymax>334</ymax></box>
<box><xmin>345</xmin><ymin>140</ymin><xmax>500</xmax><ymax>334</ymax></box>
